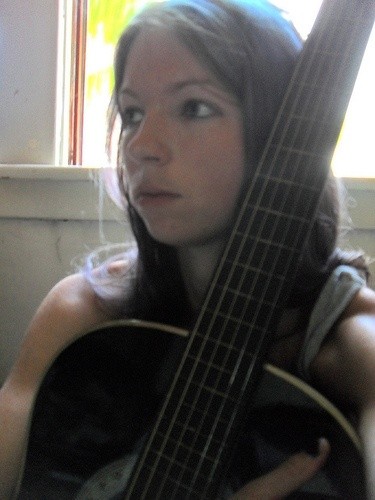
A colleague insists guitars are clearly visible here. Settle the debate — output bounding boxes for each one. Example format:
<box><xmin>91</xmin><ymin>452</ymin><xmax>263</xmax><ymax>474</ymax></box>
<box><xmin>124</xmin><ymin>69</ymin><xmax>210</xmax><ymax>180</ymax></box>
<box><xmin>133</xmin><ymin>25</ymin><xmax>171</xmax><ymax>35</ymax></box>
<box><xmin>10</xmin><ymin>0</ymin><xmax>375</xmax><ymax>499</ymax></box>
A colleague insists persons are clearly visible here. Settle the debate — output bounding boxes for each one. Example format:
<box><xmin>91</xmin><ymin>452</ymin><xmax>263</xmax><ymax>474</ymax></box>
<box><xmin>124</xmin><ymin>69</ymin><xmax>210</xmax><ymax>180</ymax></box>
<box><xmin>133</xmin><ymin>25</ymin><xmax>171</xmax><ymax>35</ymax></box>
<box><xmin>1</xmin><ymin>1</ymin><xmax>373</xmax><ymax>499</ymax></box>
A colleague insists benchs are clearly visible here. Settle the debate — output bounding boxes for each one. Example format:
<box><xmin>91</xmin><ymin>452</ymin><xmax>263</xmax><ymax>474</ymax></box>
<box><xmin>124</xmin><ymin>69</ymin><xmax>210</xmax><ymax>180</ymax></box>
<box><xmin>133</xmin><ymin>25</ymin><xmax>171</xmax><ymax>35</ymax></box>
<box><xmin>0</xmin><ymin>164</ymin><xmax>374</xmax><ymax>387</ymax></box>
